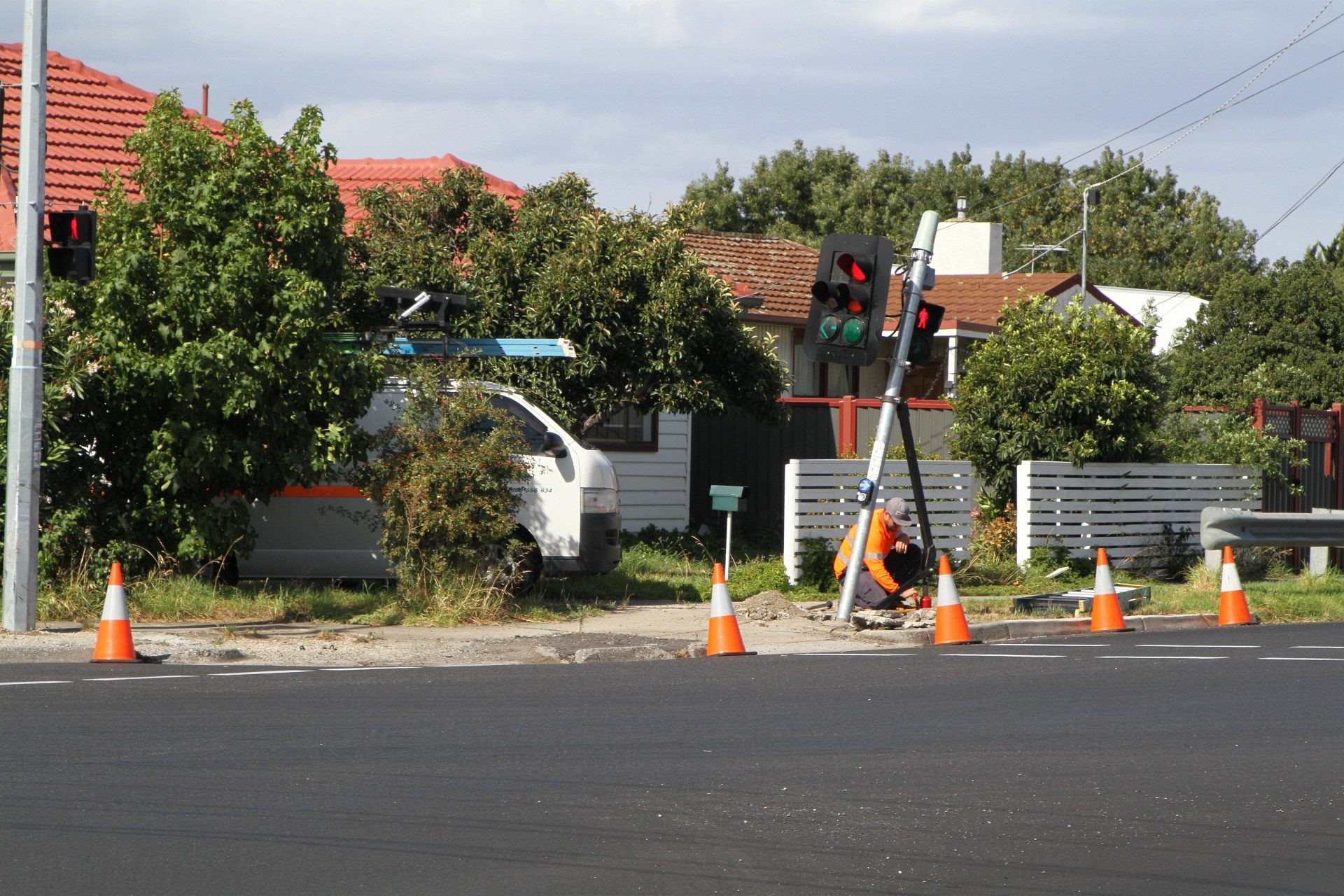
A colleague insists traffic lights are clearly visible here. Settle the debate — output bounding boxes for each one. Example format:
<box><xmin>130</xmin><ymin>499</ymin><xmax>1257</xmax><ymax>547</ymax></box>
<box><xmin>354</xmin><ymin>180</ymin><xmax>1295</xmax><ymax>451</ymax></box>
<box><xmin>48</xmin><ymin>208</ymin><xmax>98</xmax><ymax>282</ymax></box>
<box><xmin>802</xmin><ymin>233</ymin><xmax>894</xmax><ymax>365</ymax></box>
<box><xmin>909</xmin><ymin>299</ymin><xmax>945</xmax><ymax>368</ymax></box>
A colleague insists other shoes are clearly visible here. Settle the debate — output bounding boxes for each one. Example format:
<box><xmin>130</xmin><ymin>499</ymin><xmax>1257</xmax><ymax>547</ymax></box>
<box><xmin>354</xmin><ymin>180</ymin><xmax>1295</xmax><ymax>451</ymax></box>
<box><xmin>894</xmin><ymin>603</ymin><xmax>916</xmax><ymax>613</ymax></box>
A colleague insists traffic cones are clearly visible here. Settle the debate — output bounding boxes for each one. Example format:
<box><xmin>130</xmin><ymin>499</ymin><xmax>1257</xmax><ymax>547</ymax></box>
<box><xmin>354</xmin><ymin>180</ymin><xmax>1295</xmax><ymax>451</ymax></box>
<box><xmin>1210</xmin><ymin>544</ymin><xmax>1262</xmax><ymax>627</ymax></box>
<box><xmin>1086</xmin><ymin>547</ymin><xmax>1137</xmax><ymax>634</ymax></box>
<box><xmin>706</xmin><ymin>562</ymin><xmax>758</xmax><ymax>656</ymax></box>
<box><xmin>928</xmin><ymin>555</ymin><xmax>984</xmax><ymax>645</ymax></box>
<box><xmin>89</xmin><ymin>558</ymin><xmax>141</xmax><ymax>663</ymax></box>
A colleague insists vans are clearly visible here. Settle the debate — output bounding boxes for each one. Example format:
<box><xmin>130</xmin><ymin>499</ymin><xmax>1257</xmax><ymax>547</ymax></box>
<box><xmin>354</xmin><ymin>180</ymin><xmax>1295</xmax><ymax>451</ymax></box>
<box><xmin>232</xmin><ymin>379</ymin><xmax>625</xmax><ymax>602</ymax></box>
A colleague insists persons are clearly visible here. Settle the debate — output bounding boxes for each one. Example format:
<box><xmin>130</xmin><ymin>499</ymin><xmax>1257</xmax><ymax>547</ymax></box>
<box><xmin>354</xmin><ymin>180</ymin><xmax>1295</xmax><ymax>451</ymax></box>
<box><xmin>834</xmin><ymin>496</ymin><xmax>921</xmax><ymax>610</ymax></box>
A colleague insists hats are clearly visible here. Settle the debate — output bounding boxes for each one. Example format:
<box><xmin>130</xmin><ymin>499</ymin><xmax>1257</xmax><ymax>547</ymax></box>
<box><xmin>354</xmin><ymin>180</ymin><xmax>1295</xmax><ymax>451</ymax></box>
<box><xmin>885</xmin><ymin>497</ymin><xmax>912</xmax><ymax>526</ymax></box>
